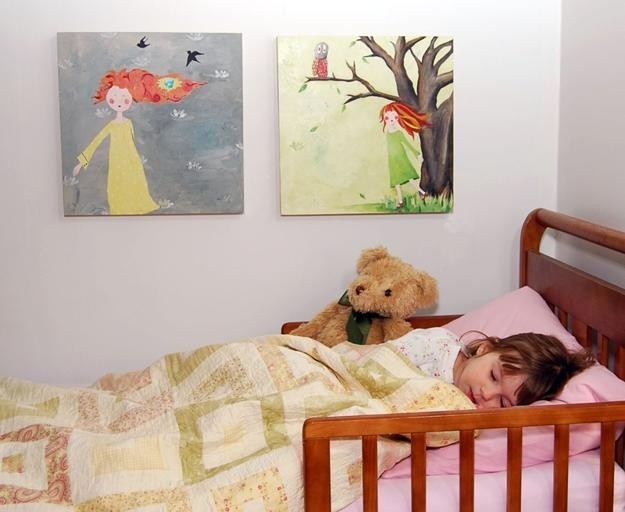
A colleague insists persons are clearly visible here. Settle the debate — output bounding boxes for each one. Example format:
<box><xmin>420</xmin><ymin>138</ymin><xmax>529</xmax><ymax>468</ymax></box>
<box><xmin>372</xmin><ymin>324</ymin><xmax>598</xmax><ymax>410</ymax></box>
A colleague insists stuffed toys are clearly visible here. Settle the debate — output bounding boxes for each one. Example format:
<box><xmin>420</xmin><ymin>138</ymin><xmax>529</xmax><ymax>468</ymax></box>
<box><xmin>285</xmin><ymin>243</ymin><xmax>438</xmax><ymax>349</ymax></box>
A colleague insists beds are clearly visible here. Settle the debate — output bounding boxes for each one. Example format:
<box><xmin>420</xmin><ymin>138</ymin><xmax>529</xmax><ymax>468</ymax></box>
<box><xmin>0</xmin><ymin>209</ymin><xmax>625</xmax><ymax>512</ymax></box>
